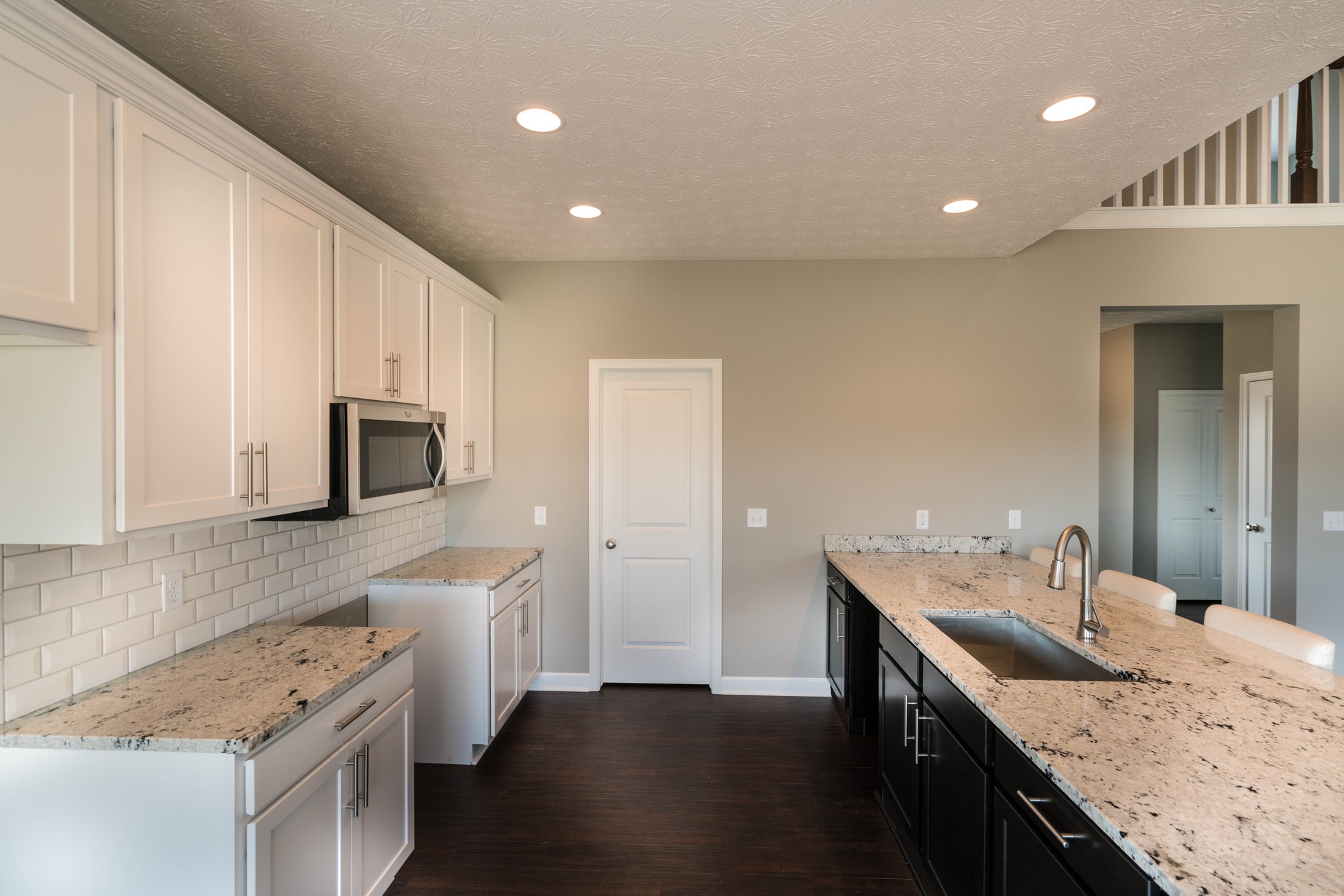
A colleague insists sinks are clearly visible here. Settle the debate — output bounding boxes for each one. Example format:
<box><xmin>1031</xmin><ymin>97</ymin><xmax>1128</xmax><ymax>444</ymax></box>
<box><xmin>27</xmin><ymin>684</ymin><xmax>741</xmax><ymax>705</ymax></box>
<box><xmin>914</xmin><ymin>606</ymin><xmax>1146</xmax><ymax>683</ymax></box>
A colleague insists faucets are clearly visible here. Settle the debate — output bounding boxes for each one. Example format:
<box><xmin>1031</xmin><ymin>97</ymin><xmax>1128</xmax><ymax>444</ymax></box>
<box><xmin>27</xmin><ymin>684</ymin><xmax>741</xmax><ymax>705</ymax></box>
<box><xmin>1047</xmin><ymin>526</ymin><xmax>1110</xmax><ymax>643</ymax></box>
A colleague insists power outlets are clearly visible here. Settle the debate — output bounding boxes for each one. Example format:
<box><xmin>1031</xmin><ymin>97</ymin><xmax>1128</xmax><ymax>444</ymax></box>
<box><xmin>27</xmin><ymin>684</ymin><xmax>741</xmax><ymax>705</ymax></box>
<box><xmin>917</xmin><ymin>510</ymin><xmax>929</xmax><ymax>529</ymax></box>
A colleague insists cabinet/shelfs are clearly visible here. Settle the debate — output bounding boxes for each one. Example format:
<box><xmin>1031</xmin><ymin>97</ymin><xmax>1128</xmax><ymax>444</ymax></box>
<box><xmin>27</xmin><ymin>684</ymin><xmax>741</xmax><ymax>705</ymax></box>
<box><xmin>331</xmin><ymin>225</ymin><xmax>428</xmax><ymax>406</ymax></box>
<box><xmin>428</xmin><ymin>280</ymin><xmax>493</xmax><ymax>486</ymax></box>
<box><xmin>0</xmin><ymin>23</ymin><xmax>98</xmax><ymax>343</ymax></box>
<box><xmin>875</xmin><ymin>614</ymin><xmax>991</xmax><ymax>896</ymax></box>
<box><xmin>826</xmin><ymin>561</ymin><xmax>880</xmax><ymax>735</ymax></box>
<box><xmin>1</xmin><ymin>648</ymin><xmax>415</xmax><ymax>896</ymax></box>
<box><xmin>367</xmin><ymin>558</ymin><xmax>542</xmax><ymax>765</ymax></box>
<box><xmin>1</xmin><ymin>100</ymin><xmax>332</xmax><ymax>548</ymax></box>
<box><xmin>991</xmin><ymin>723</ymin><xmax>1157</xmax><ymax>896</ymax></box>
